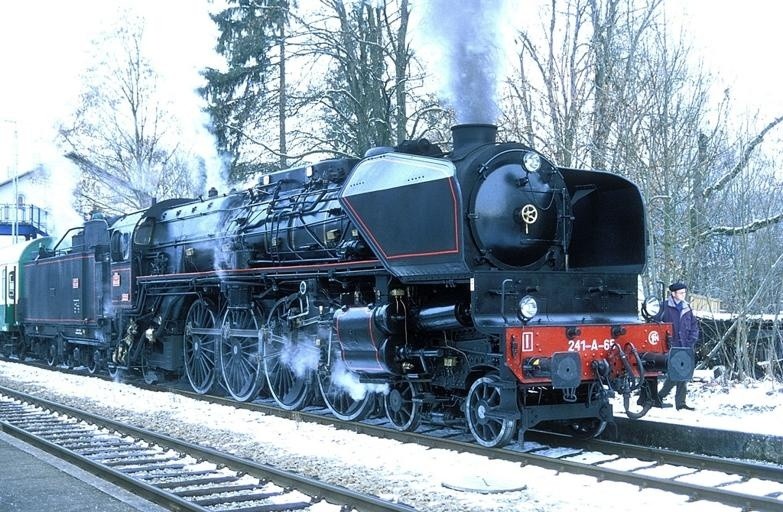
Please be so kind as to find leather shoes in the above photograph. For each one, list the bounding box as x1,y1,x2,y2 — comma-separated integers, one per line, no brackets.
677,405,695,411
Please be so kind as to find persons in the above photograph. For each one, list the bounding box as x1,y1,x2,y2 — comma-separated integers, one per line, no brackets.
653,283,700,411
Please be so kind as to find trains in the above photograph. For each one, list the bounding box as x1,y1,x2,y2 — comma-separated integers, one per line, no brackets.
0,125,696,450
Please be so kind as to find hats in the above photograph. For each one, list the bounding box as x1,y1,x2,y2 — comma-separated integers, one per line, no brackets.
669,283,687,292
